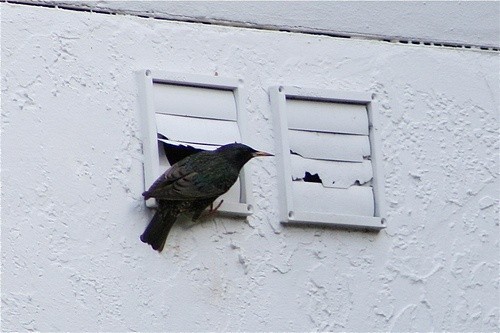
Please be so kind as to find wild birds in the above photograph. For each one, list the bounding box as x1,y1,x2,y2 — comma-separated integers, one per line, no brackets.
140,133,276,253
